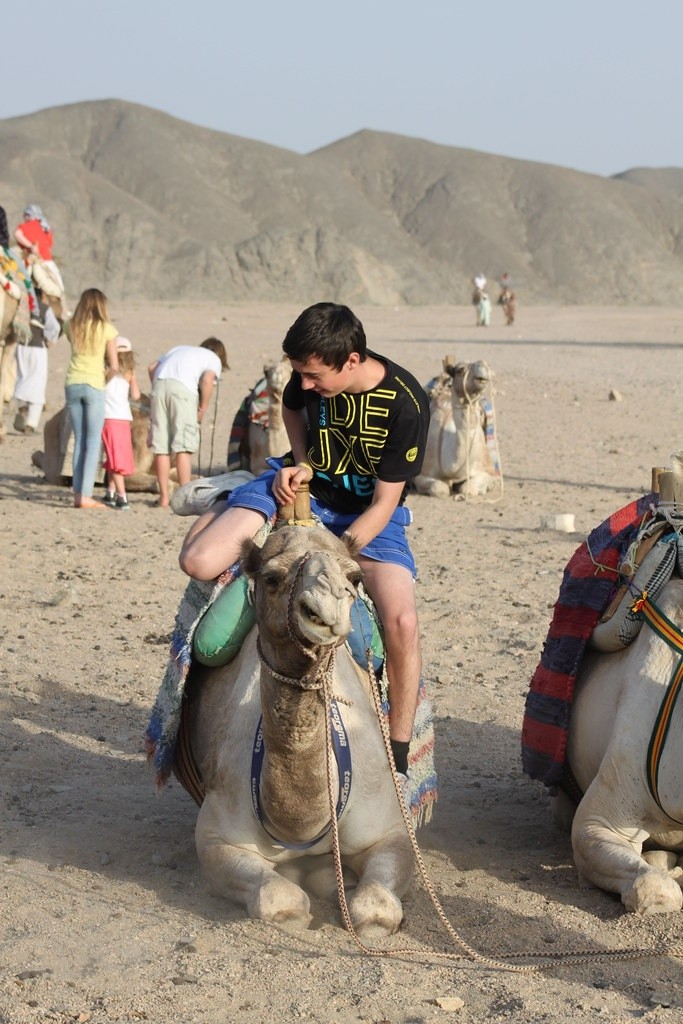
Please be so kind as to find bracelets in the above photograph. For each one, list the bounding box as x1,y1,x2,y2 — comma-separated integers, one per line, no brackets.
298,461,314,484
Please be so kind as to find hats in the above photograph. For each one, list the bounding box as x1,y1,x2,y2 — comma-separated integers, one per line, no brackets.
115,335,132,353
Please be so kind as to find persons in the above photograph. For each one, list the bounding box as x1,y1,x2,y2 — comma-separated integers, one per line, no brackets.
0,205,232,510
471,273,518,326
168,302,430,775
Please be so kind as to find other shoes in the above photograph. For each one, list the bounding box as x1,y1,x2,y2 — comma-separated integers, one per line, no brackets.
100,496,117,507
12,410,25,432
23,426,41,436
116,499,130,512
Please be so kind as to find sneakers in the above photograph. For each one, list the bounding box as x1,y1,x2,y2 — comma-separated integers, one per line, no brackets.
169,469,257,517
395,770,414,809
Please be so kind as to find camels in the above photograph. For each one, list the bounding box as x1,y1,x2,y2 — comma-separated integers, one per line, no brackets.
471,289,517,326
0,248,65,445
412,361,490,501
165,519,416,941
33,391,207,507
242,354,294,477
568,496,683,919
